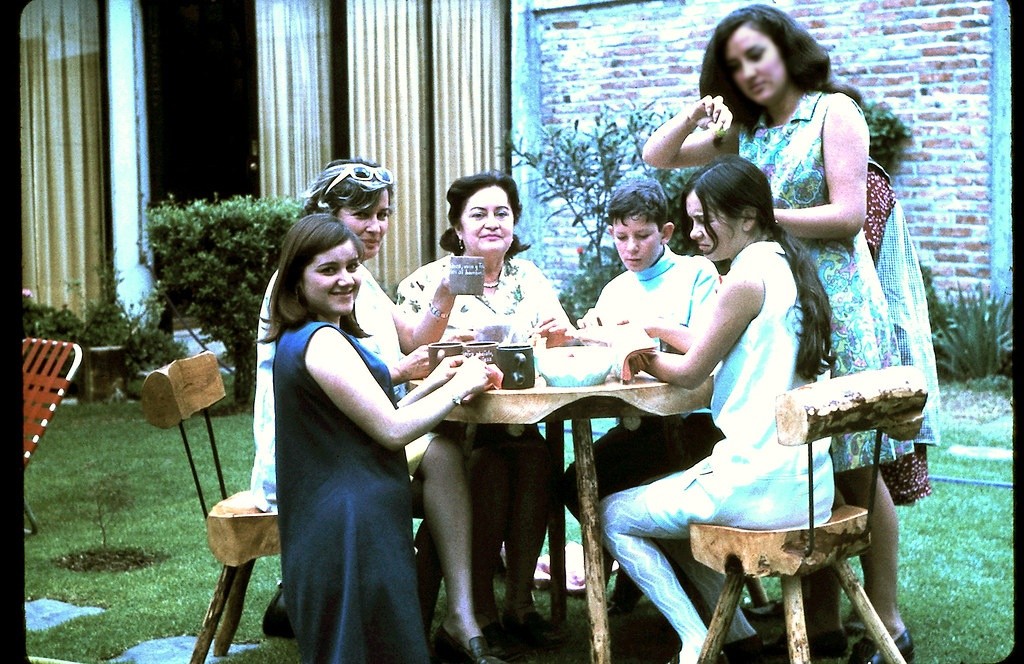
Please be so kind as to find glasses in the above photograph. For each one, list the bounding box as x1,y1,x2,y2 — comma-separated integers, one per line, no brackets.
323,163,395,194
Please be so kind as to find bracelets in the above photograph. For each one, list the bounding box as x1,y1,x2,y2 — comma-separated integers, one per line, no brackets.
443,384,462,404
430,305,450,318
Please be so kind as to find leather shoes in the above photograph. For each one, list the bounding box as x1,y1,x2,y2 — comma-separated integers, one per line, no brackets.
849,630,914,664
432,623,509,664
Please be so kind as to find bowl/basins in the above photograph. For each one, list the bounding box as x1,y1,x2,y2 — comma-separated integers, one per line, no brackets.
536,346,614,386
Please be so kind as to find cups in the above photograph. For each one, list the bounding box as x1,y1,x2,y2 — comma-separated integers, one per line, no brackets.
496,345,536,390
428,341,462,374
448,256,485,296
463,342,498,366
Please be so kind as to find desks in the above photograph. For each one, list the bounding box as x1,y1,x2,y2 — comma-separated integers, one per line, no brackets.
408,371,714,663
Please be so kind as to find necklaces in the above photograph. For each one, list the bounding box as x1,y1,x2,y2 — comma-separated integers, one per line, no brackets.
483,282,497,288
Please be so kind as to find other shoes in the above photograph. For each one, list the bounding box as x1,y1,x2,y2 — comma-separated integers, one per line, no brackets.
503,609,565,649
723,630,765,662
769,628,849,662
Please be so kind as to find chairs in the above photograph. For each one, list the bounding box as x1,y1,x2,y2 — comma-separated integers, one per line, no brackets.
22,337,82,536
141,350,281,664
689,365,928,664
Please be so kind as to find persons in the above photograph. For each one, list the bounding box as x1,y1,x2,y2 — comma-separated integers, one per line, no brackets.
251,159,509,664
393,168,582,661
564,180,721,652
642,4,913,664
599,153,835,664
